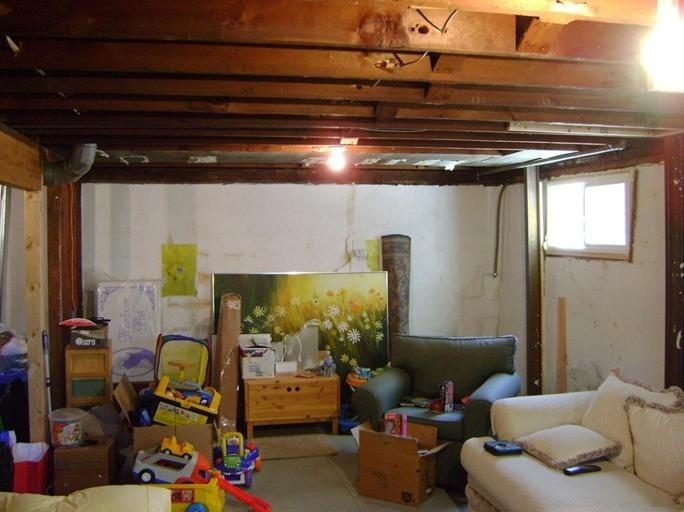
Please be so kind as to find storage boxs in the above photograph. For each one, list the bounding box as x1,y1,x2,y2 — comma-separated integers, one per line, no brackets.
114,373,220,466
71,323,109,347
239,346,274,379
351,419,450,507
48,408,85,447
54,436,116,496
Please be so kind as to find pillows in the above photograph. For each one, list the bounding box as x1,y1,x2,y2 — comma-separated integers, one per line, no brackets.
515,424,622,471
623,394,684,505
579,369,684,469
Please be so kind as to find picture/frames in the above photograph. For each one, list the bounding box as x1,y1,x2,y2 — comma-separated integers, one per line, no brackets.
211,271,389,384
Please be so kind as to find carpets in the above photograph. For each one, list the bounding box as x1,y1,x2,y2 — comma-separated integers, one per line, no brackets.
248,425,338,459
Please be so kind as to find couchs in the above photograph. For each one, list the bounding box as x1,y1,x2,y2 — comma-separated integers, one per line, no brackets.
351,332,521,490
462,370,683,512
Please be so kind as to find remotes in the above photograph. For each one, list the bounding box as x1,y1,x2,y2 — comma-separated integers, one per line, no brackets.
563,464,601,476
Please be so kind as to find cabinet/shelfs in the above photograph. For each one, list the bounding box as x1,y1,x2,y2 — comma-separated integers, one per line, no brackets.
244,370,339,440
65,339,111,408
